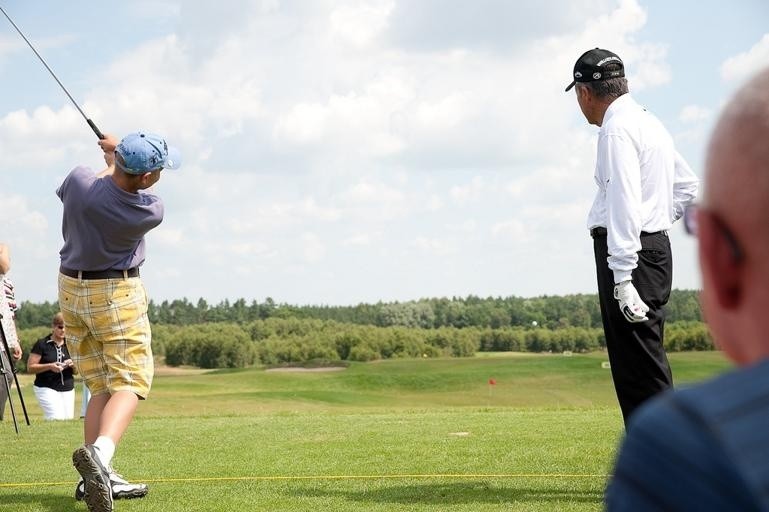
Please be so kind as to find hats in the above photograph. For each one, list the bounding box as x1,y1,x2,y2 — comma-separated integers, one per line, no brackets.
565,48,625,90
113,129,182,175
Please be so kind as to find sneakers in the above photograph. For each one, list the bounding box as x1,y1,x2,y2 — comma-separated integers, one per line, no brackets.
72,445,115,511
76,469,148,501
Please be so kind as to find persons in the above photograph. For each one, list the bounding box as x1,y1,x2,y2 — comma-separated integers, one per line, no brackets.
0,243,24,421
25,311,79,422
594,67,768,512
562,47,699,440
54,129,183,512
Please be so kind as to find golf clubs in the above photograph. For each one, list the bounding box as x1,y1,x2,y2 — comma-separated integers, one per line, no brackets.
533,321,537,326
489,378,496,384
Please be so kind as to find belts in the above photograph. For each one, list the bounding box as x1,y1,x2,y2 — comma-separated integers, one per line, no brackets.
590,228,605,238
58,266,139,279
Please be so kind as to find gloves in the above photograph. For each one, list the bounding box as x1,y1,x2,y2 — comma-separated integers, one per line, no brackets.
614,281,650,322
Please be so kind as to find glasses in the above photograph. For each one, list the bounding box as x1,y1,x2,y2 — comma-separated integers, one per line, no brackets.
57,326,64,329
682,205,742,263
